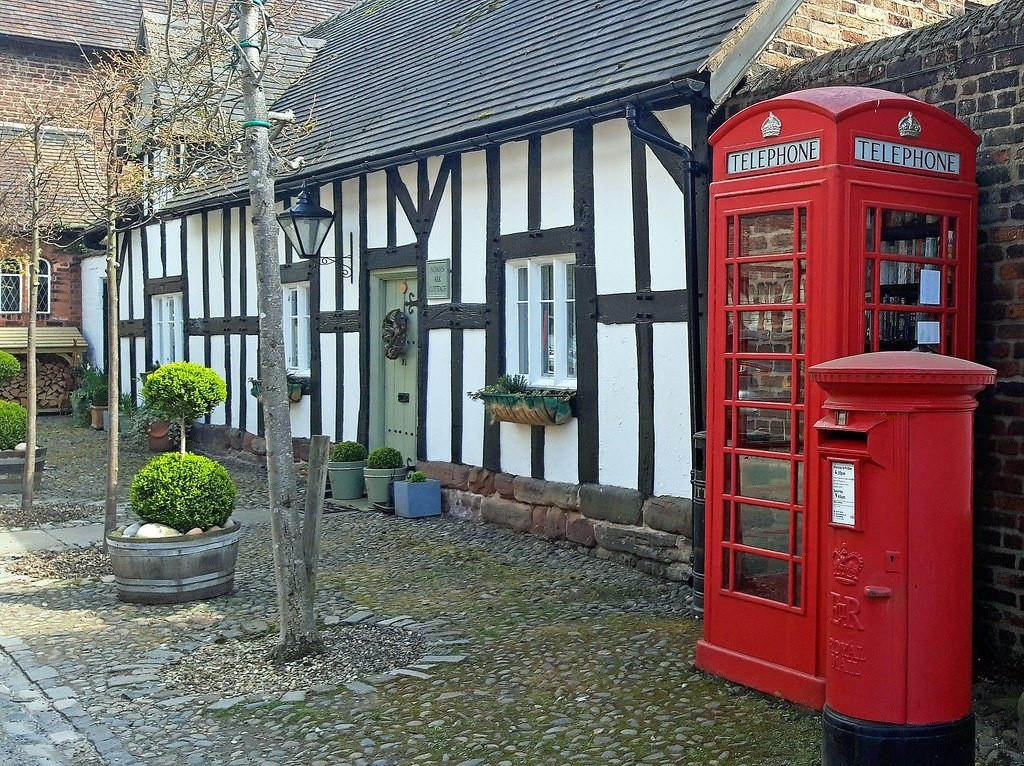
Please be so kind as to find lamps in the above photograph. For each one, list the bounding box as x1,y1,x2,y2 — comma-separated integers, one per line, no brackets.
275,185,353,284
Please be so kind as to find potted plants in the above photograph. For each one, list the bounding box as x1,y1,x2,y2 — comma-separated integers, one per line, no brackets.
394,471,441,518
105,451,240,605
0,400,47,493
76,364,177,450
328,440,367,500
363,446,407,502
